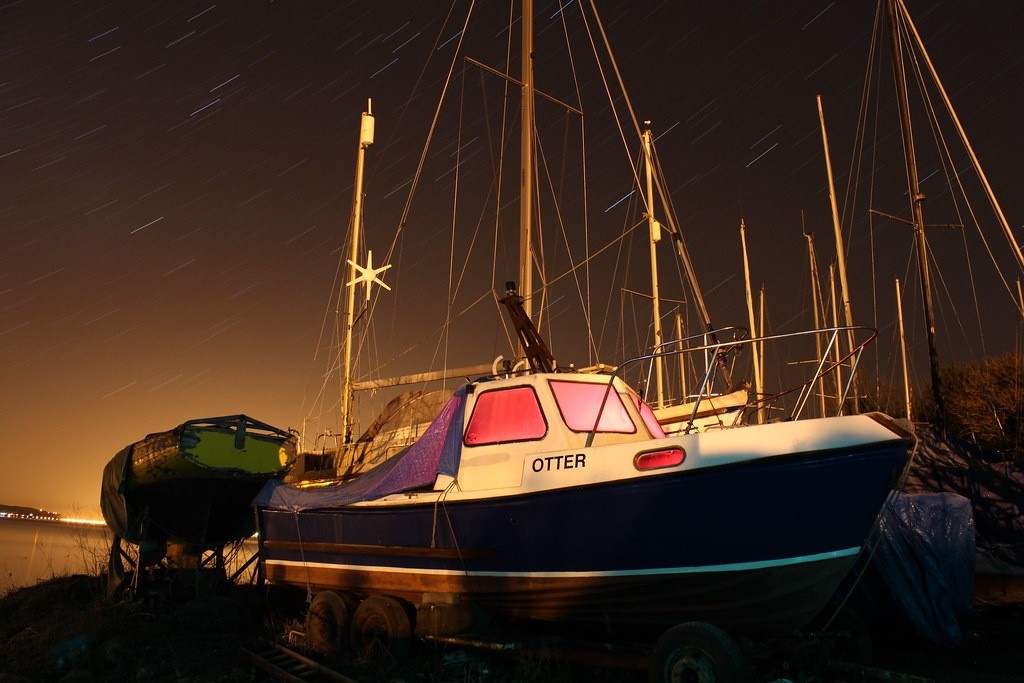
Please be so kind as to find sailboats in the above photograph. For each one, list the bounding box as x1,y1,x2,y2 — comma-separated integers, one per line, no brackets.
97,1,916,640
